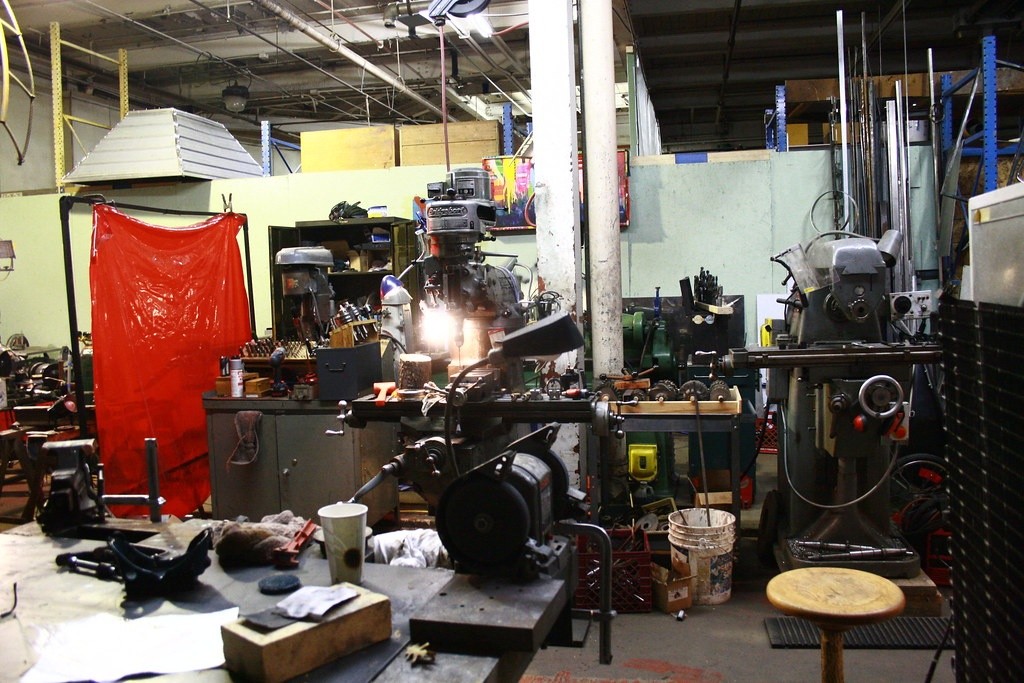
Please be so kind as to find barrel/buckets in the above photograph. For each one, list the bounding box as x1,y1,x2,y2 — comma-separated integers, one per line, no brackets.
667,507,737,606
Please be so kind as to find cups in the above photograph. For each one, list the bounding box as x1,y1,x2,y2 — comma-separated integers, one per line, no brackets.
317,502,368,587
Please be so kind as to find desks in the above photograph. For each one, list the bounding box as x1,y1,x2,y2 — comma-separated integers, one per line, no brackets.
0,520,541,683
351,391,598,522
584,415,744,578
12,346,62,360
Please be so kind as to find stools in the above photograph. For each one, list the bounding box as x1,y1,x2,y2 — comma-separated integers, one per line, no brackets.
766,567,905,683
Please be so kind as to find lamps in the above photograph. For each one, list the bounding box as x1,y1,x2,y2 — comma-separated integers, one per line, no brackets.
222,79,250,112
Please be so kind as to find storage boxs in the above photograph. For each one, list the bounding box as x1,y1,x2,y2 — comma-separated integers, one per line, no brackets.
322,239,350,259
345,250,374,271
694,469,733,511
755,411,778,454
574,528,653,614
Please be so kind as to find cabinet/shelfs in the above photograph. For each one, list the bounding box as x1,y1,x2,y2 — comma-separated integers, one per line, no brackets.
267,217,426,341
202,389,401,528
